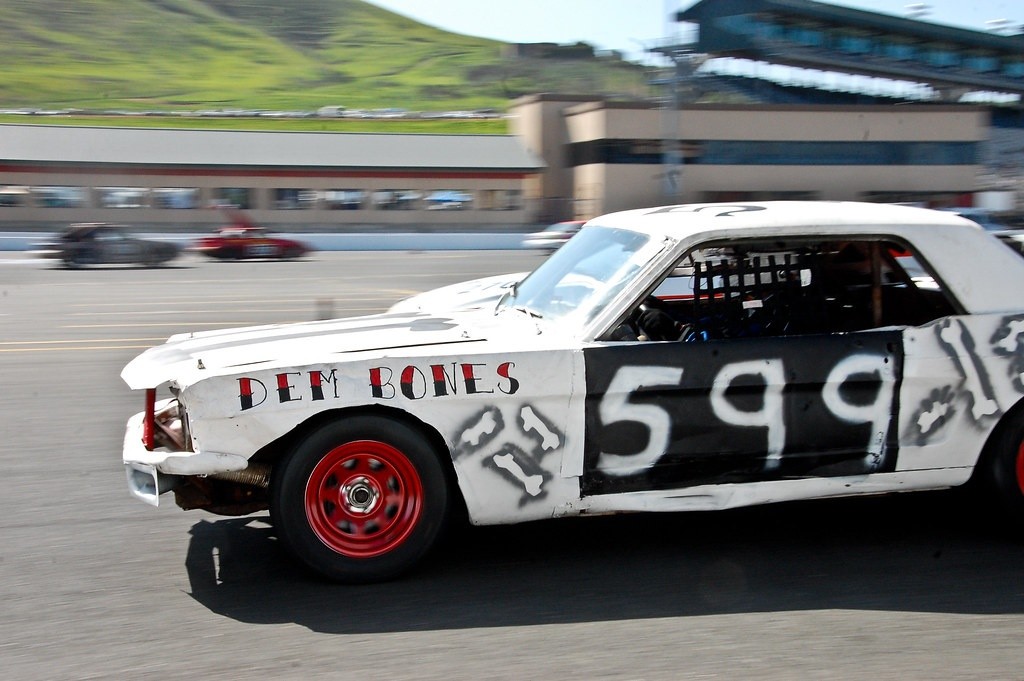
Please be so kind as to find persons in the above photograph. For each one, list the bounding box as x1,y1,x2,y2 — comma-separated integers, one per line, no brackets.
638,242,814,340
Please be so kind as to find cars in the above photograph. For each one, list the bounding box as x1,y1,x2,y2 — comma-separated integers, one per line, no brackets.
26,219,184,268
118,198,1024,590
182,202,313,260
384,205,1024,311
520,219,590,255
